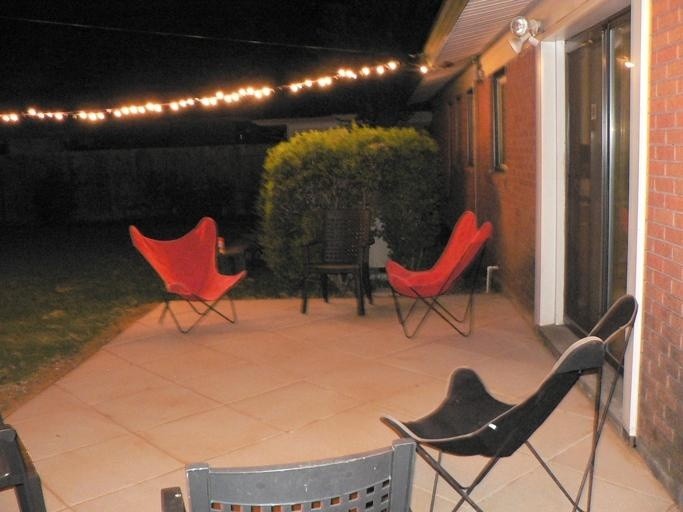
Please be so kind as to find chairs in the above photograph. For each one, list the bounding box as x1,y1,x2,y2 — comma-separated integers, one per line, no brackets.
301,207,373,317
0,415,46,512
379,293,639,512
385,211,494,339
128,217,247,334
161,438,417,512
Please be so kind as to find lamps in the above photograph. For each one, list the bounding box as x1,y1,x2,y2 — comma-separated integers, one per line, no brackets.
506,16,542,55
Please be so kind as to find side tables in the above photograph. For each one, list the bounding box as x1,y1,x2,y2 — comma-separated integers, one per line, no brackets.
214,245,245,275
